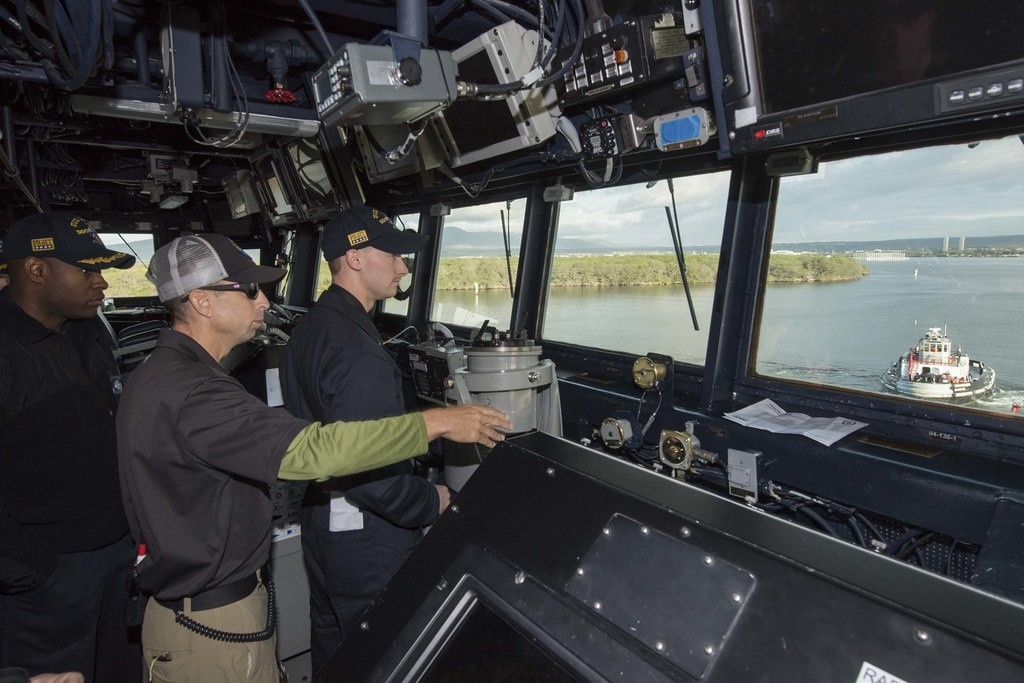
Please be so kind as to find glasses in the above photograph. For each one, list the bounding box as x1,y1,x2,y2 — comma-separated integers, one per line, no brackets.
180,282,259,303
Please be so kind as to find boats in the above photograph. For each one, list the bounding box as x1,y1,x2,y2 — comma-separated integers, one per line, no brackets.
879,320,995,398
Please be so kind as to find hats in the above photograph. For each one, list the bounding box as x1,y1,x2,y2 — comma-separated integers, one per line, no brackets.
1,210,136,272
319,206,431,261
145,231,288,304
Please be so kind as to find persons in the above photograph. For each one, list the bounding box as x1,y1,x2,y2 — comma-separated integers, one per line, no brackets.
116,233,514,683
0,211,136,683
278,206,450,681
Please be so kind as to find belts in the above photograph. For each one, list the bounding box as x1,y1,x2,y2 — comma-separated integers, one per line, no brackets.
153,569,264,612
